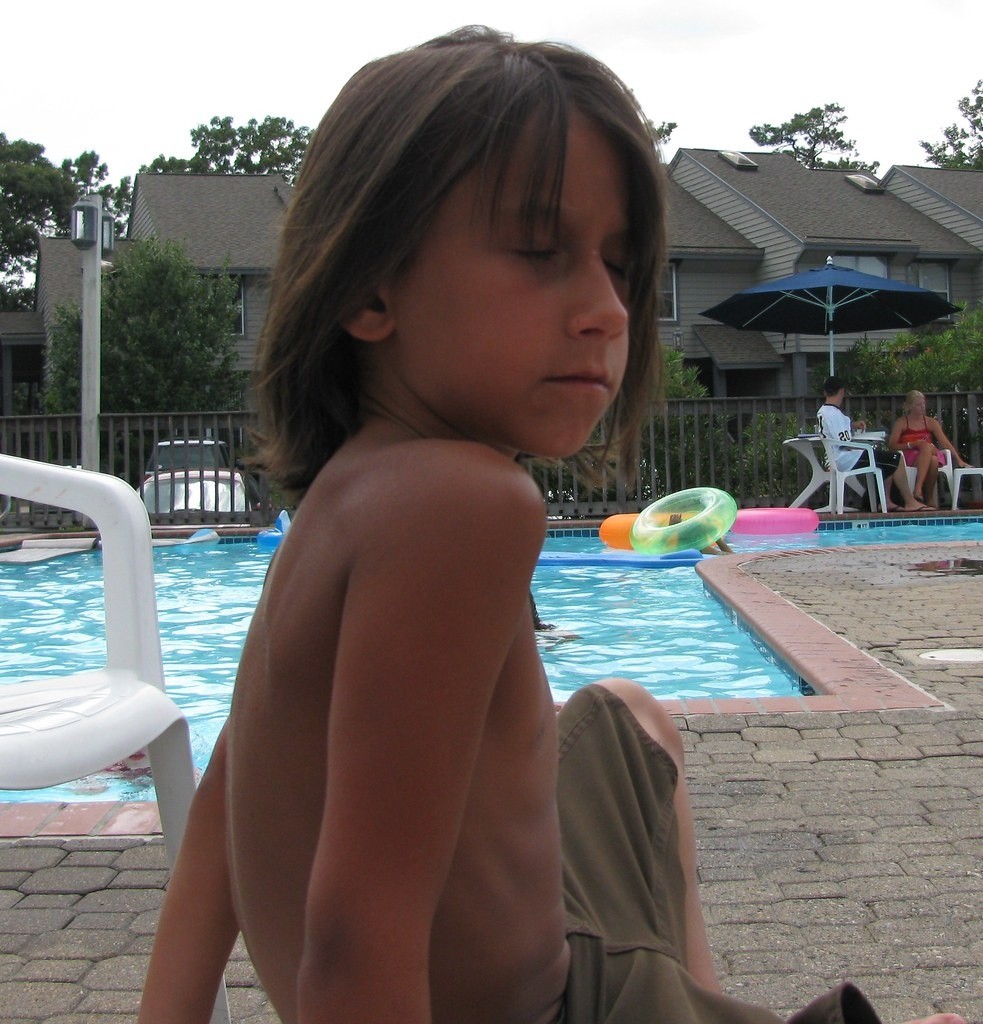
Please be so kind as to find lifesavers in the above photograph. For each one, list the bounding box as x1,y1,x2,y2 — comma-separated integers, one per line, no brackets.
728,508,820,534
598,487,737,555
256,510,292,550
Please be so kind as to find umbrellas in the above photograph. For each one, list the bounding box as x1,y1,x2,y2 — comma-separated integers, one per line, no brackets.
697,256,965,377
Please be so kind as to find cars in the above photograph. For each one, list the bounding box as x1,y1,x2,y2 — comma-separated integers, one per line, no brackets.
135,469,274,530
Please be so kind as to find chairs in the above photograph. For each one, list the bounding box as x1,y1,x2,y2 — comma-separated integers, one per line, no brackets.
898,447,953,499
820,438,887,514
0,448,234,1024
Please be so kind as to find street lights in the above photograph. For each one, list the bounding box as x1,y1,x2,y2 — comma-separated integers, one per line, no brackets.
71,191,118,526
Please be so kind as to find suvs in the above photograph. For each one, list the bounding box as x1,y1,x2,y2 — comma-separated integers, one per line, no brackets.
145,439,244,479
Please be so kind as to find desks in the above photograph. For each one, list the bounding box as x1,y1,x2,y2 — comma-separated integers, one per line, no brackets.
783,437,885,508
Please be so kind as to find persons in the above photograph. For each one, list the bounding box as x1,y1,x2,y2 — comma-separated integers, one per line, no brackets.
817,375,937,511
143,24,965,1024
887,390,974,510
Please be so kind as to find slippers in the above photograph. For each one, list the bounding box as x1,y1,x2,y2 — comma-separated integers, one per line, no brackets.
903,505,937,512
913,490,923,500
878,504,905,512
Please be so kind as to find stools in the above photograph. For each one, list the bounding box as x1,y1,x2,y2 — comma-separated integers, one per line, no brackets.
952,467,983,511
